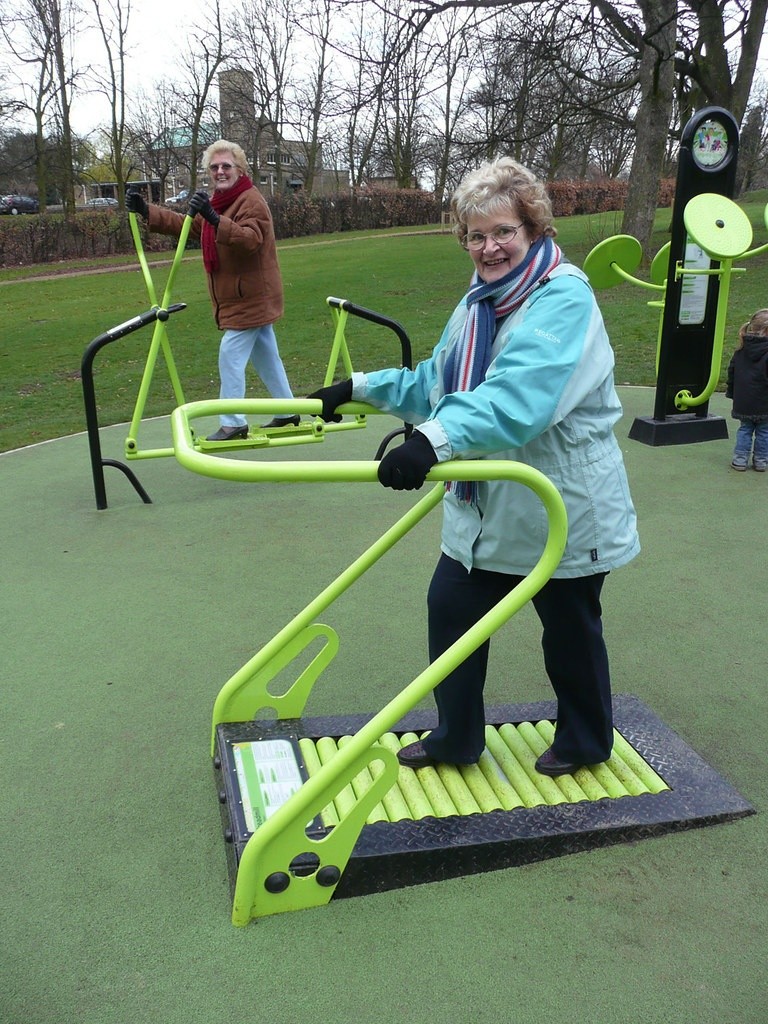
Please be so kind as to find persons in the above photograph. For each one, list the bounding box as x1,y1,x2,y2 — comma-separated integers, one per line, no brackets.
124,140,301,441
308,155,642,775
725,308,768,472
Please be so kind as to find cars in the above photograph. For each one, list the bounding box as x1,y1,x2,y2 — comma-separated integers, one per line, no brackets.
86,198,117,205
0,194,40,216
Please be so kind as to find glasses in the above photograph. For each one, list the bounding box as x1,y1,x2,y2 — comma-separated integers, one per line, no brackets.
461,222,526,251
209,163,238,172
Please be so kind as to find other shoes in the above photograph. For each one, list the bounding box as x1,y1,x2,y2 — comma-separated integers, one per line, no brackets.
732,463,747,471
535,745,581,775
396,739,485,766
756,466,765,472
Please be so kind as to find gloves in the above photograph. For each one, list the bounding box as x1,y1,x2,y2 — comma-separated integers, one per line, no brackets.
307,377,353,422
188,191,219,225
124,189,148,219
378,432,437,491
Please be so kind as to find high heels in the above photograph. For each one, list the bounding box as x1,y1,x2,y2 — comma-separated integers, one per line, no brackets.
261,414,300,428
206,424,249,441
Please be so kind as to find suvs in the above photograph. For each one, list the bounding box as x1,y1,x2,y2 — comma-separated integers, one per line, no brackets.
165,189,209,203
